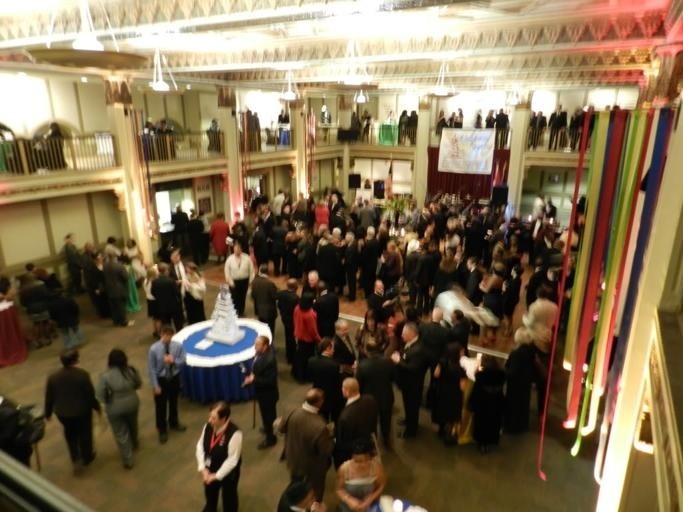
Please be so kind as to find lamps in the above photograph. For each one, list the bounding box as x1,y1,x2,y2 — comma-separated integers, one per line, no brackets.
72,0,103,51
281,71,299,101
353,86,372,106
428,64,458,97
148,50,172,93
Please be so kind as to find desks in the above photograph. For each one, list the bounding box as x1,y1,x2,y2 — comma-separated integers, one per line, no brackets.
167,316,267,407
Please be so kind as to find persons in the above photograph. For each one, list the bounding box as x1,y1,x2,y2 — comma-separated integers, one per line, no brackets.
435,107,508,148
145,120,173,134
251,112,262,152
350,110,372,144
527,104,620,153
397,108,418,146
277,109,290,145
194,376,386,510
210,120,217,131
170,190,621,453
45,123,62,138
0,232,206,476
